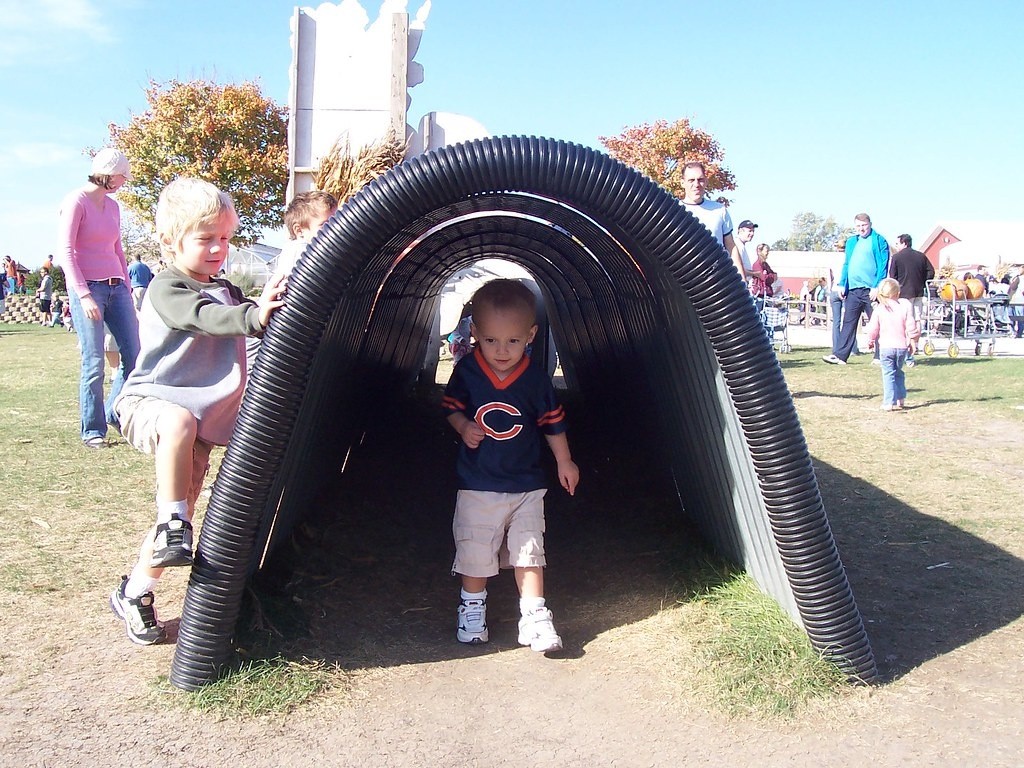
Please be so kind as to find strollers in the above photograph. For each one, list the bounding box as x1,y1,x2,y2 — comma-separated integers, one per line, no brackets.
760,287,792,355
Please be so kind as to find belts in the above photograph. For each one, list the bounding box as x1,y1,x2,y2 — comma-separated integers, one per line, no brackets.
100,277,123,286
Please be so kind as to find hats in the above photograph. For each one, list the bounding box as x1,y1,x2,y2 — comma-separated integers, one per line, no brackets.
738,219,758,229
93,149,136,183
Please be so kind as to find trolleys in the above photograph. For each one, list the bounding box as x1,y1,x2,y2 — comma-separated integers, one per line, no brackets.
923,279,1002,358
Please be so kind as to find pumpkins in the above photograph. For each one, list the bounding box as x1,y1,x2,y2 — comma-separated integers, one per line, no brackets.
964,279,984,299
940,278,969,300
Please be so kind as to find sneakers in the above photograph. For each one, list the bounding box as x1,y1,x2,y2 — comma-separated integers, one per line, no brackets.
822,354,846,365
109,574,168,646
150,511,195,568
517,597,562,651
871,358,880,365
456,588,489,644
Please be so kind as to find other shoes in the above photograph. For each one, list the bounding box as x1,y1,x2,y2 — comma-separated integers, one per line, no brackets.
87,438,106,449
897,399,903,407
879,403,891,410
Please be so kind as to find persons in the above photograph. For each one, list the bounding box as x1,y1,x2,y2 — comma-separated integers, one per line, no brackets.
800,278,827,326
889,234,935,355
937,264,1024,338
822,213,890,365
114,177,338,646
866,278,919,411
678,163,783,300
442,279,579,651
0,255,73,333
60,147,154,449
